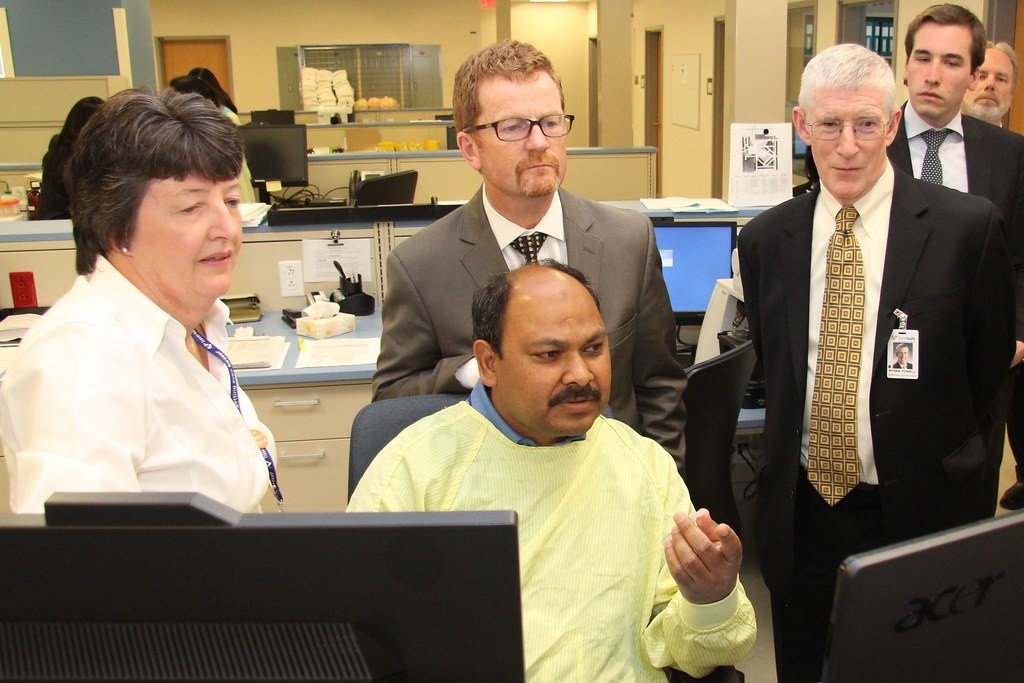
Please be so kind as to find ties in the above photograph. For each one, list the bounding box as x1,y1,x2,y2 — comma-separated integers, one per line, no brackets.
806,207,865,506
509,233,547,265
921,130,949,189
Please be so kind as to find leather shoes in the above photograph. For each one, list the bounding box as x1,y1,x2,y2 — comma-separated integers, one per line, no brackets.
999,481,1024,510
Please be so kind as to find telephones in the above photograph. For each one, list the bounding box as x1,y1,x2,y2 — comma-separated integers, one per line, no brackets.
350,169,385,199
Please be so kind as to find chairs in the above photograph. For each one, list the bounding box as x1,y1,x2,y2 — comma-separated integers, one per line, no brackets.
684,340,758,537
349,394,614,511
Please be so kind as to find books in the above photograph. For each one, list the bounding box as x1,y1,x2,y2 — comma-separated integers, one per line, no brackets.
0,314,43,343
227,336,284,368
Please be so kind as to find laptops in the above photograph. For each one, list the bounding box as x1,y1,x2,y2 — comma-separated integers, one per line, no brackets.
819,508,1023,683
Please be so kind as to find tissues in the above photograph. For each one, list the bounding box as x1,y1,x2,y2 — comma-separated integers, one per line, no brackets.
296,300,356,340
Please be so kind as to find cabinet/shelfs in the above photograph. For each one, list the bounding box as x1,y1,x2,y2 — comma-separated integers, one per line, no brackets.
238,379,373,513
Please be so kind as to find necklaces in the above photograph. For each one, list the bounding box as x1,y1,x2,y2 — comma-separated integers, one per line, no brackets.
185,329,208,369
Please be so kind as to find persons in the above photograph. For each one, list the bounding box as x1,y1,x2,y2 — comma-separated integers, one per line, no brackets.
35,68,256,220
738,44,1024,683
893,344,912,368
371,40,686,477
346,258,757,683
0,84,278,514
885,4,1024,221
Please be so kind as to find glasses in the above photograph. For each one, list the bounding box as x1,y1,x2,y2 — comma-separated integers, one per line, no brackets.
807,110,892,141
462,112,575,143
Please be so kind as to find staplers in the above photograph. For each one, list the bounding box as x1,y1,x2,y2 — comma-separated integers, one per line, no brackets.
281,308,302,329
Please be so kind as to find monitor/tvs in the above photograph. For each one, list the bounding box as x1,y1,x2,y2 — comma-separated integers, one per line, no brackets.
0,491,524,683
250,110,294,124
237,123,308,205
435,115,454,120
653,220,737,325
447,126,459,149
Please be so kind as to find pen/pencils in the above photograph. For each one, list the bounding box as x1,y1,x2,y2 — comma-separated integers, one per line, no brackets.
333,261,347,280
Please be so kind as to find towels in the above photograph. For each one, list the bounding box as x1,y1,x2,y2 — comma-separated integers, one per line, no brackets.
300,66,356,110
